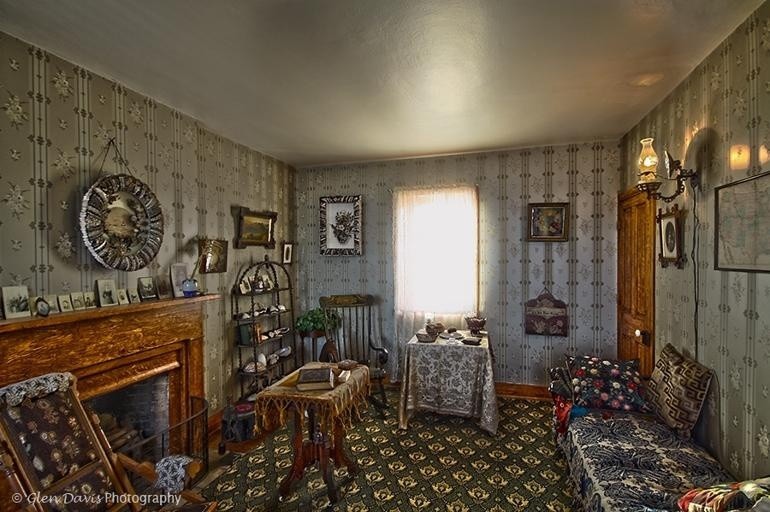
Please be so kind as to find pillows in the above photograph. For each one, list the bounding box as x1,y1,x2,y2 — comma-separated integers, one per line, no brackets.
547,340,716,435
659,471,770,512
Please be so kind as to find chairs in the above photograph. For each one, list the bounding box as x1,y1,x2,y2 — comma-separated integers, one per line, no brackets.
0,372,218,511
318,294,390,421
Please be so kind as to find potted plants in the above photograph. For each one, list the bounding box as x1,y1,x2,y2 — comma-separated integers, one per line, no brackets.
294,306,340,338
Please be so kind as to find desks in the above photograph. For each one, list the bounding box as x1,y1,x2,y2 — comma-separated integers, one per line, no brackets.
406,327,490,419
260,360,369,500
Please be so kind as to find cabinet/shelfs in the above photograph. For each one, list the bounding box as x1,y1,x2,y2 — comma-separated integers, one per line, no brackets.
232,254,299,399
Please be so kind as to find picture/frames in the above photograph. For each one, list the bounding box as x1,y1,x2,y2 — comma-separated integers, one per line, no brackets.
317,193,363,256
237,206,295,266
527,201,570,242
655,203,686,270
713,172,769,274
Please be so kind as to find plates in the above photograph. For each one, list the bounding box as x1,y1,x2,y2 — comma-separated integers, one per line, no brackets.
440,332,464,338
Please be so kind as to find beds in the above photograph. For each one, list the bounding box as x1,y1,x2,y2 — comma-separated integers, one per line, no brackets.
548,375,757,511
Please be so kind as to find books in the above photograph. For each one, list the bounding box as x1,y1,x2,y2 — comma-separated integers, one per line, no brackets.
295,367,335,393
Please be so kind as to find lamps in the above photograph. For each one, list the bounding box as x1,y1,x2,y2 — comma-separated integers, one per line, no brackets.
636,137,699,203
